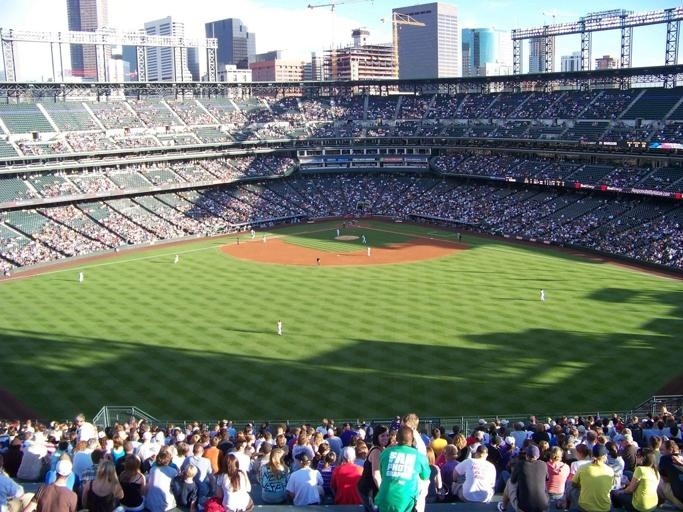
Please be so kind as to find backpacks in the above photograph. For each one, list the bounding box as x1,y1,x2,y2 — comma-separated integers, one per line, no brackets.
197,482,226,512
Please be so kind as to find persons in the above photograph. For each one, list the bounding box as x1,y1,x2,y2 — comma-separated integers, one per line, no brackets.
1,403,683,512
0,89,683,279
539,288,545,301
79,271,84,282
276,319,283,335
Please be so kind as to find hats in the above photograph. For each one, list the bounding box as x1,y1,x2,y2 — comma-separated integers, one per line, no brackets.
474,431,485,440
141,431,152,440
505,436,515,445
176,433,185,441
443,445,459,455
587,430,598,439
295,452,310,463
592,445,609,456
156,432,164,440
525,445,540,458
344,447,356,459
611,418,618,423
618,434,633,442
98,431,106,438
500,420,509,425
56,460,72,476
479,419,487,424
